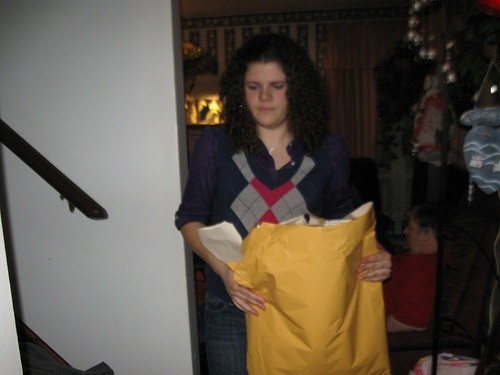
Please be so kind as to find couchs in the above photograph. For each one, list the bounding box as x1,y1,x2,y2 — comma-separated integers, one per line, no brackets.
388,201,500,375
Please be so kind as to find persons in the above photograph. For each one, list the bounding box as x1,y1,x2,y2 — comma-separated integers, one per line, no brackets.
174,33,393,375
383,204,439,332
